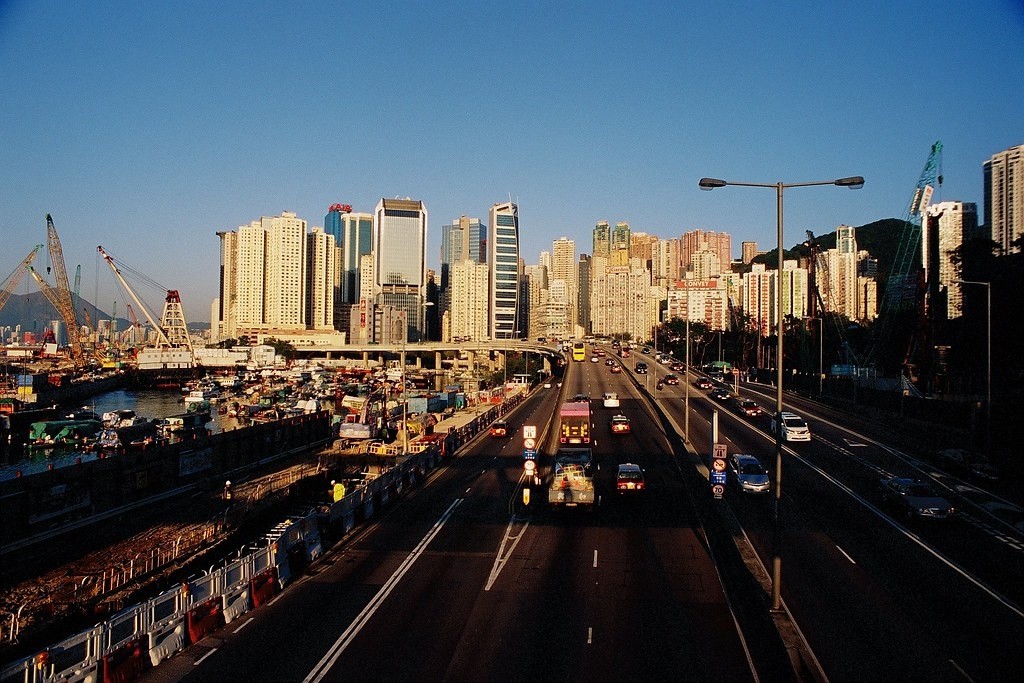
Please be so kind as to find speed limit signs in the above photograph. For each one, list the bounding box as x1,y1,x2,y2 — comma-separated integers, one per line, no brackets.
524,461,535,470
713,484,724,494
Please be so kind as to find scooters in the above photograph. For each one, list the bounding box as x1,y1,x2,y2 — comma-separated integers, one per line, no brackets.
657,383,663,390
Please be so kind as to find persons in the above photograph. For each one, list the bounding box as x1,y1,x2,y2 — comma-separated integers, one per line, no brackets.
36,646,56,682
331,480,345,502
222,481,234,499
270,540,278,564
310,505,330,514
146,435,152,442
182,580,189,599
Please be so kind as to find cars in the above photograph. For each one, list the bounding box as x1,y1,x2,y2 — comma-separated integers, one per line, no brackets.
544,341,650,374
613,463,646,496
664,374,678,384
736,399,762,417
712,388,730,400
610,415,631,435
881,479,956,524
490,419,509,436
654,351,671,364
602,392,620,407
671,362,682,370
695,379,713,389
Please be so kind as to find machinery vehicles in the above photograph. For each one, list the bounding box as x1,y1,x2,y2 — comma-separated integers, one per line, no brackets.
339,385,387,442
806,140,944,402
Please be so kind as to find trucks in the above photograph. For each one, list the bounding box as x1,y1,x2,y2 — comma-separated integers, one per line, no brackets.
548,394,595,510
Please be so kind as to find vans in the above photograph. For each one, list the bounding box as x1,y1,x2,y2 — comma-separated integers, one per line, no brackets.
771,412,811,442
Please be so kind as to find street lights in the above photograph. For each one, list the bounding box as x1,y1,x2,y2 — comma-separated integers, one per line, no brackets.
475,324,500,417
401,302,434,455
504,331,521,399
699,176,866,609
654,274,721,444
951,279,991,406
801,316,823,395
747,324,760,369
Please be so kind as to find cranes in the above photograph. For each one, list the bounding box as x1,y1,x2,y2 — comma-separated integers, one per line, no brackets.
96,245,191,350
0,213,145,366
